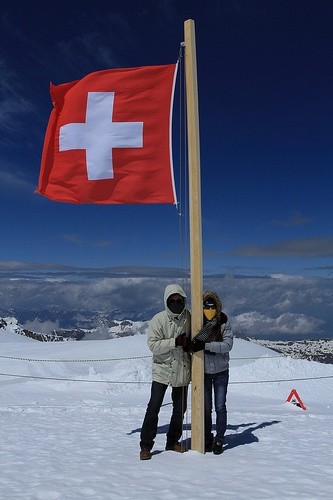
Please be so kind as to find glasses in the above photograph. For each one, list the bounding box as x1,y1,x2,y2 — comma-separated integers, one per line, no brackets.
166,298,184,304
204,303,217,309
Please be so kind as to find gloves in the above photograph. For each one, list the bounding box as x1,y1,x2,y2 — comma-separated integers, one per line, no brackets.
175,333,188,346
190,339,205,352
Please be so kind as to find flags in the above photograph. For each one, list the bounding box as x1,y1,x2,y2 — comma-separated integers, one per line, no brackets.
34,64,179,204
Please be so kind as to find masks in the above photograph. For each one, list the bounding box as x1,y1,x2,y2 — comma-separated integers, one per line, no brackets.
168,304,185,314
203,308,217,320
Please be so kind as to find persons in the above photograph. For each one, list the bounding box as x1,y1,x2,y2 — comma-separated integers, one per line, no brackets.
193,291,233,455
140,284,192,460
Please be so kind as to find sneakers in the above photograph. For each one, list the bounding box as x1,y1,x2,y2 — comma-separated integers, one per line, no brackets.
166,441,188,452
140,446,151,460
205,433,214,451
212,441,223,454
